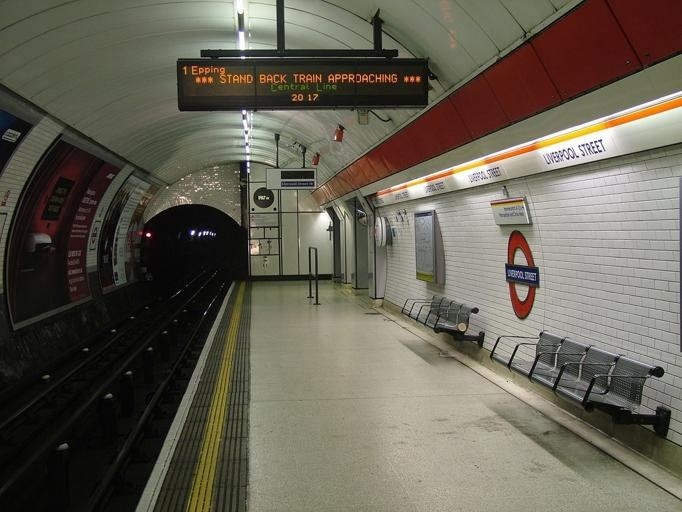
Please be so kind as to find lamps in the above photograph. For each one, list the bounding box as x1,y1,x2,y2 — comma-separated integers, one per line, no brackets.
311,152,321,165
236,1,251,175
330,123,345,142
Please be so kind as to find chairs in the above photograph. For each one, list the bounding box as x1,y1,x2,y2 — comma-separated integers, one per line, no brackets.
402,293,443,325
529,344,619,395
489,329,565,372
505,337,590,382
409,295,451,318
417,300,456,327
425,303,485,349
554,356,671,437
